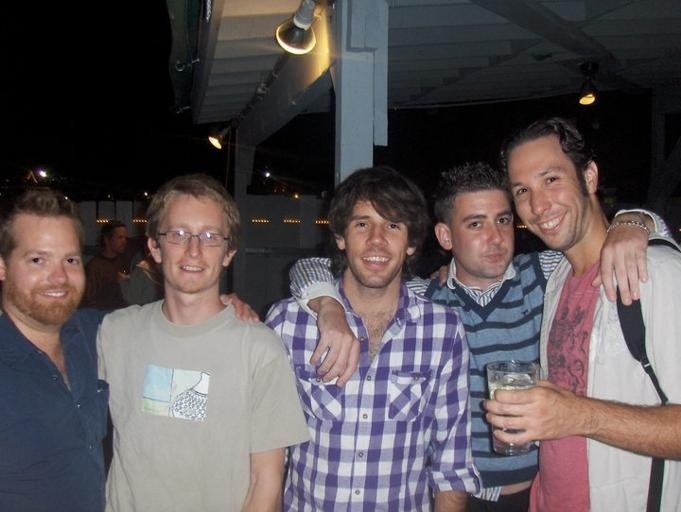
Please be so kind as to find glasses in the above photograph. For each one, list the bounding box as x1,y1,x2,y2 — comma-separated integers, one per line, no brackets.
156,229,231,247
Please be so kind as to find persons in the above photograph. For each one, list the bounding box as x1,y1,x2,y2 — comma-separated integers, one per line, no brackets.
289,161,673,512
86,220,127,311
95,172,311,512
428,118,681,511
0,186,262,511
116,240,163,307
265,166,482,512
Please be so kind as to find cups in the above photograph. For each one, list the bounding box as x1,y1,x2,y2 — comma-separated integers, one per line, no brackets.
486,360,540,456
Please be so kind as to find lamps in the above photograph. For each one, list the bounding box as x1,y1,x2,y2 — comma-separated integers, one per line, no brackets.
578,63,598,105
176,59,199,71
208,127,230,150
275,0,316,55
174,104,190,114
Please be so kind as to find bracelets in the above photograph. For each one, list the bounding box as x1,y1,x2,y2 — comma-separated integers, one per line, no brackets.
607,220,650,233
